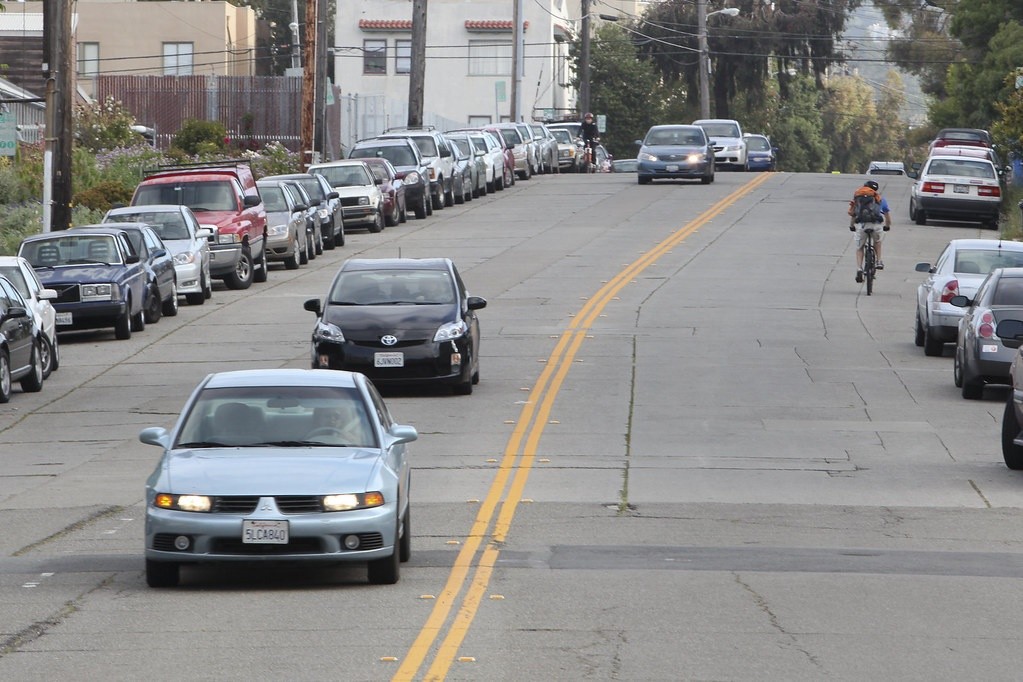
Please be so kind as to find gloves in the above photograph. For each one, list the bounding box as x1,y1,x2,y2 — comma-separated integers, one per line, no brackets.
849,225,855,232
883,225,890,231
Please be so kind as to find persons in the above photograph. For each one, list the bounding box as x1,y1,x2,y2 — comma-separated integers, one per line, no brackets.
311,407,342,436
413,277,451,301
850,181,891,282
576,113,600,168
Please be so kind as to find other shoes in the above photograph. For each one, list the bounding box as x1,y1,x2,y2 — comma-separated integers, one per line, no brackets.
876,260,884,269
855,269,864,283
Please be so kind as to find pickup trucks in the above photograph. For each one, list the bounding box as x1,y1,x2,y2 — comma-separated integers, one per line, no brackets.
128,158,268,289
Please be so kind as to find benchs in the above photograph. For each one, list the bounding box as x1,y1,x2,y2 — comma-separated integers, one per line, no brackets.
956,260,979,273
206,410,313,438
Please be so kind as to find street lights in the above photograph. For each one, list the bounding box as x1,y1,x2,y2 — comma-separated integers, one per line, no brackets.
698,8,740,121
289,22,301,69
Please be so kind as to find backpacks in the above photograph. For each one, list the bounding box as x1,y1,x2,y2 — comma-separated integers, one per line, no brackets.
854,195,884,223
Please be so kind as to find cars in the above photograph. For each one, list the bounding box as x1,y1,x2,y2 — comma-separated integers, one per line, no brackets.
915,239,1022,357
0,256,59,370
949,267,1023,400
303,257,488,396
255,157,408,269
17,227,148,341
634,119,779,186
996,319,1023,470
0,273,44,404
100,204,212,306
866,129,1013,231
138,368,418,588
47,221,179,324
442,122,638,205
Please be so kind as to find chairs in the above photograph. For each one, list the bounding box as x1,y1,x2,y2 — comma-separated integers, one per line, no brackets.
417,143,432,153
348,173,363,187
263,191,285,212
311,406,352,435
165,216,185,238
38,245,60,266
88,239,111,261
214,402,257,441
348,277,380,300
392,149,407,165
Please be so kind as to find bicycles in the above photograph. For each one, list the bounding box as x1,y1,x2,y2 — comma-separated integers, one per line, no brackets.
576,138,597,174
852,229,889,296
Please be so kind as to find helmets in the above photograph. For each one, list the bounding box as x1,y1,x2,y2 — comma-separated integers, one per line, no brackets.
865,181,878,190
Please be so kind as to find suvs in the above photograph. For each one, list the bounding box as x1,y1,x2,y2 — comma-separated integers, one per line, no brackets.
347,126,455,219
